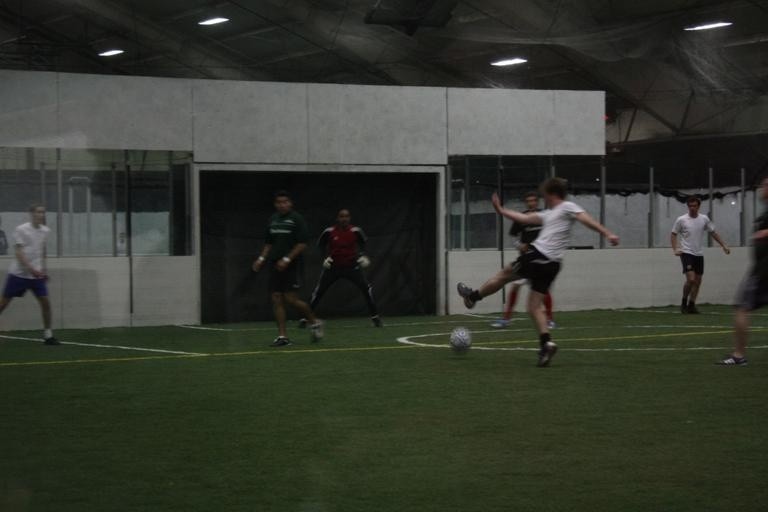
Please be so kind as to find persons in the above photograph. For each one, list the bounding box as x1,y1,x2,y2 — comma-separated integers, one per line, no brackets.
249,191,324,348
457,175,620,367
490,192,557,332
715,174,768,364
295,203,384,331
0,202,60,346
669,196,732,314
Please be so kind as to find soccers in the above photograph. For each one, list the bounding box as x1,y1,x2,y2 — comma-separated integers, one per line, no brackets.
451,328,473,348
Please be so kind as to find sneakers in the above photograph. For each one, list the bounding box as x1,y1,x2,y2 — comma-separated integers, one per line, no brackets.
713,354,747,366
371,314,383,326
300,316,323,339
680,304,699,314
457,282,475,308
268,336,292,347
43,336,60,346
538,341,557,367
491,318,510,328
